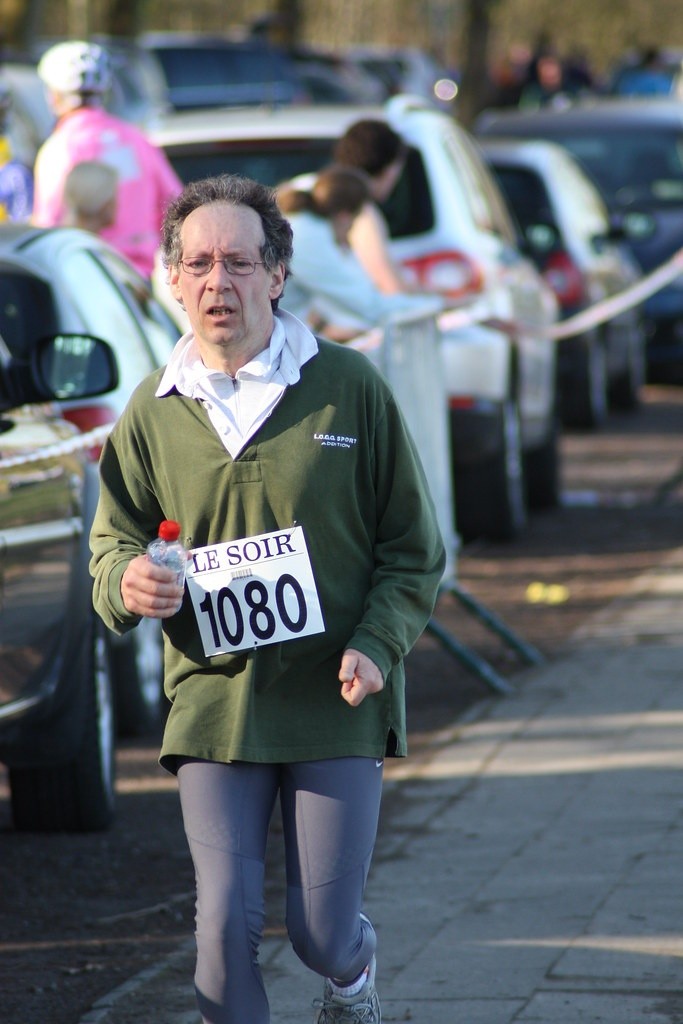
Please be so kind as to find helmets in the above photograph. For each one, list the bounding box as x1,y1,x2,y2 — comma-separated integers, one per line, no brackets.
36,39,114,92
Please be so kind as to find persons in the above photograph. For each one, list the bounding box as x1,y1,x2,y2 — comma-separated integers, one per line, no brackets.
0,41,189,278
518,44,672,116
273,119,445,346
89,175,448,1024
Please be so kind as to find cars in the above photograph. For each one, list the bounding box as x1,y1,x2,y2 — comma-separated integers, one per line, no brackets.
0,224,191,838
0,27,683,595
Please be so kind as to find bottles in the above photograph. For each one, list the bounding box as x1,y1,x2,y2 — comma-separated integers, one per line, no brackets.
147,520,188,613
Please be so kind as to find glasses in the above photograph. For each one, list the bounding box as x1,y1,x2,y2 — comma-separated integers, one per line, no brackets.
178,256,265,275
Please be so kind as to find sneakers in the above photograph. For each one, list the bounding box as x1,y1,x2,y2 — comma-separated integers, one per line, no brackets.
311,911,381,1024
